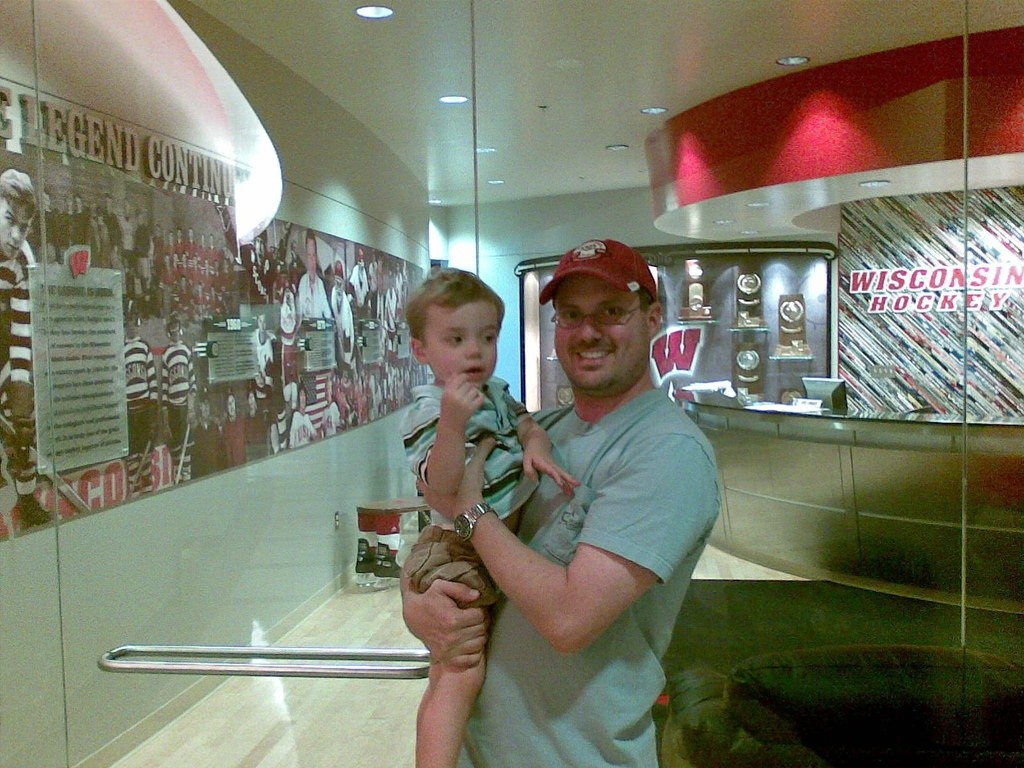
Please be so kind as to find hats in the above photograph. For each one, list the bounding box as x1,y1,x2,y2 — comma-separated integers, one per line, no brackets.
226,388,235,399
539,240,657,305
247,383,256,396
199,387,210,402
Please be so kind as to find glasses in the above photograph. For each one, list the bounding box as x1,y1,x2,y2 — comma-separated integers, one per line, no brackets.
551,302,642,330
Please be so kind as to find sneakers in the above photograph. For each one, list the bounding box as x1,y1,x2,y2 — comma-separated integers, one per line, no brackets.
354,538,377,585
373,541,403,589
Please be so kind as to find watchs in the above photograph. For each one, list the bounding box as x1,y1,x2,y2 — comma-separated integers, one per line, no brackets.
452,502,499,541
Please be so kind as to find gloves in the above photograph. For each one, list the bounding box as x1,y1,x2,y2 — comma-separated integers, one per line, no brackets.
186,408,197,424
162,423,172,441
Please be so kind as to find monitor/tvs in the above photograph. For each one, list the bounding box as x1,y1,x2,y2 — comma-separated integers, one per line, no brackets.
801,376,849,411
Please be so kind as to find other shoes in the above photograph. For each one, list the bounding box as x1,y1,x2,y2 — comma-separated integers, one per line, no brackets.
17,492,48,524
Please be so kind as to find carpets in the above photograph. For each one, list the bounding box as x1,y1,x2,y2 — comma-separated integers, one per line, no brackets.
652,580,1024,768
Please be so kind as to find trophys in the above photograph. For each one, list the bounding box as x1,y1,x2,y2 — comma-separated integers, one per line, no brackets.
551,260,816,411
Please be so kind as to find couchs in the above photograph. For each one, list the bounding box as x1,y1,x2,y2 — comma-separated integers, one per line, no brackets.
660,644,1024,768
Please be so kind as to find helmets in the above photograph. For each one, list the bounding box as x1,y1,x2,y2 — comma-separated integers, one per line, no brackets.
123,311,142,327
334,261,343,283
358,249,364,261
254,231,267,247
165,311,182,342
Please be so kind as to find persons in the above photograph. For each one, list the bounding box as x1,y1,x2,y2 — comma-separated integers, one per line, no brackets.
242,230,424,456
400,268,581,768
398,240,723,768
0,169,249,525
